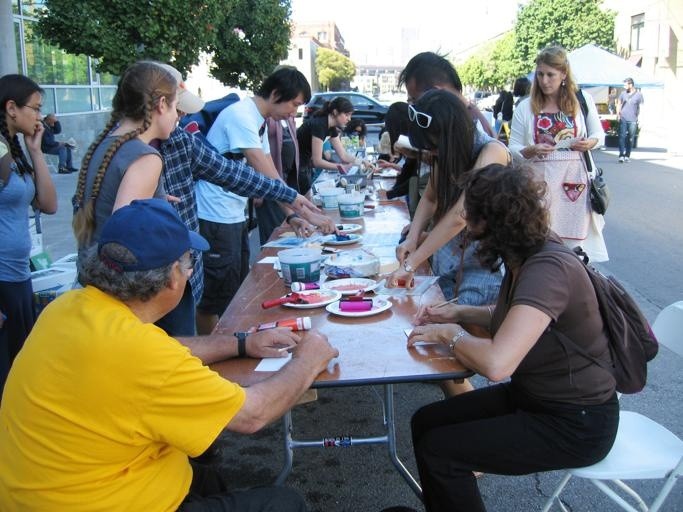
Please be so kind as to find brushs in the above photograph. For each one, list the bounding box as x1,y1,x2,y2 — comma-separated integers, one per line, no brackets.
331,229,352,241
338,287,373,312
261,290,309,309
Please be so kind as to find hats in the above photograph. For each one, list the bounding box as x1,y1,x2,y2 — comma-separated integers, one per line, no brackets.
156,63,205,114
99,199,210,271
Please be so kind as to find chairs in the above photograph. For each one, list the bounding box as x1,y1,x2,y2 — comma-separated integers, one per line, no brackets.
536,297,681,512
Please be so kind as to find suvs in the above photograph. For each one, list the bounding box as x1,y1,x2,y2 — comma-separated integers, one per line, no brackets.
300,88,395,128
468,89,492,106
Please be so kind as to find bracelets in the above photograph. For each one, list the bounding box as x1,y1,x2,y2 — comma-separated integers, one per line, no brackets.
232,331,249,358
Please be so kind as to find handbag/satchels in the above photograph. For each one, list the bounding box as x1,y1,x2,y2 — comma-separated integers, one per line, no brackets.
590,174,609,215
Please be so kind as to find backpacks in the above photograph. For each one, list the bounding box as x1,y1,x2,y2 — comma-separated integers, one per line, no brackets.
525,243,658,394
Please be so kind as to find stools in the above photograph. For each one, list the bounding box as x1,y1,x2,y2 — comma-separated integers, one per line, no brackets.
40,150,59,173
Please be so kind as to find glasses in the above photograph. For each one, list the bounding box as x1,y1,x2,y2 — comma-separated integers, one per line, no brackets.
408,104,434,128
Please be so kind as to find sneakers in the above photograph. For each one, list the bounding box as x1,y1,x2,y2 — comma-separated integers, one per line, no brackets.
59,167,78,173
619,157,629,161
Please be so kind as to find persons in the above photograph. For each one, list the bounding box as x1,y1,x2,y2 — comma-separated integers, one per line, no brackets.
40,114,78,174
70,46,619,509
0,198,340,510
616,78,644,163
0,73,57,403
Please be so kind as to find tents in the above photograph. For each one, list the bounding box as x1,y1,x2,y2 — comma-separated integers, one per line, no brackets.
527,45,664,87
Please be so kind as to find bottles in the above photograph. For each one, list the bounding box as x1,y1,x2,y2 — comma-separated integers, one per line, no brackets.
351,131,360,148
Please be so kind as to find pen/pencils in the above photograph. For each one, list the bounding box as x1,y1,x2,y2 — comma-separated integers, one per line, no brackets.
431,296,459,310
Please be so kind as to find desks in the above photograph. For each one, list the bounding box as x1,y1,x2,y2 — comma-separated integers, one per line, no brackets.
203,227,480,507
280,143,416,233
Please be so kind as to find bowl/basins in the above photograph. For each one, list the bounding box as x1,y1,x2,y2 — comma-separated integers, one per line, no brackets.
323,249,381,280
275,248,323,289
318,186,344,211
337,192,368,220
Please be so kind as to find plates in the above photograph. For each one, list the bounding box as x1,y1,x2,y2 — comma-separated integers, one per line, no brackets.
278,288,340,310
332,223,363,232
320,277,376,294
326,298,393,318
316,233,363,246
